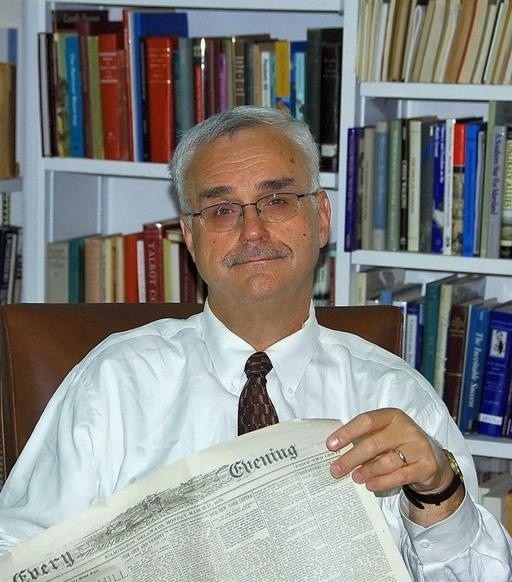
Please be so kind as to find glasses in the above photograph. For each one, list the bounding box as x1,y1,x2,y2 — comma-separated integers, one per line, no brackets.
188,192,314,234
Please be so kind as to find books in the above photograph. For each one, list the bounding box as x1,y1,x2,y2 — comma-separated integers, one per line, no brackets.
45,217,209,303
351,268,512,439
344,102,512,260
477,473,512,539
311,242,336,307
355,2,512,86
0,28,26,304
36,1,342,173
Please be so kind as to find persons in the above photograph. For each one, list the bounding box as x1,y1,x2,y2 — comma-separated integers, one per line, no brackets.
0,103,512,582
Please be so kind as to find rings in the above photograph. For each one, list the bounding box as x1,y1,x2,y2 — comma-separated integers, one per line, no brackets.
392,447,410,468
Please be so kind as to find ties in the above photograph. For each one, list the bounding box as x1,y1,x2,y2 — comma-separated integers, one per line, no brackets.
235,349,279,436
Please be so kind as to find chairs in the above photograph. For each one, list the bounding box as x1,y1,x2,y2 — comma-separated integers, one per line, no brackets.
0,303,405,493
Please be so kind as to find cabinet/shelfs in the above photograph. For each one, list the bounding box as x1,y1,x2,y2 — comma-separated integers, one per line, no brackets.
351,0,512,471
19,0,360,307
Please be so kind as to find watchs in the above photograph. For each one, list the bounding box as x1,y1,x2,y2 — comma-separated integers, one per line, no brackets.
400,447,464,511
